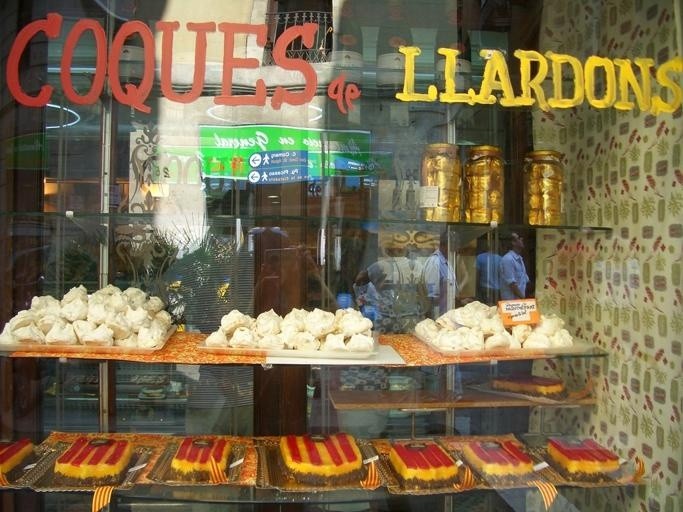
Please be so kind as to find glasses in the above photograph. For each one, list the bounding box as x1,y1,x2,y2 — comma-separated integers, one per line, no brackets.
245,226,289,242
513,235,520,242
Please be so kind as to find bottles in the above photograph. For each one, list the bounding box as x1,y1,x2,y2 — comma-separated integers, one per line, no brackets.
520,149,567,229
433,1,472,96
463,142,508,227
417,142,464,224
329,1,364,99
374,1,413,100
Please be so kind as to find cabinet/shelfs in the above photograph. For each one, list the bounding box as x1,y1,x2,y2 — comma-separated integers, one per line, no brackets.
0,69,649,512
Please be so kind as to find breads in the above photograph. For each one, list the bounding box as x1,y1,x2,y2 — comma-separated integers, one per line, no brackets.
1,284,173,350
206,307,376,354
414,300,573,353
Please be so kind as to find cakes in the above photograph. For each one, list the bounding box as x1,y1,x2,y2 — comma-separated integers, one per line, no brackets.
275,432,367,486
171,435,236,483
1,437,40,485
387,441,459,490
53,436,133,488
544,435,622,482
460,440,539,485
491,373,571,400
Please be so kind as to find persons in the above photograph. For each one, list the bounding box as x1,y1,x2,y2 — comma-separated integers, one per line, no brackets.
198,230,531,436
